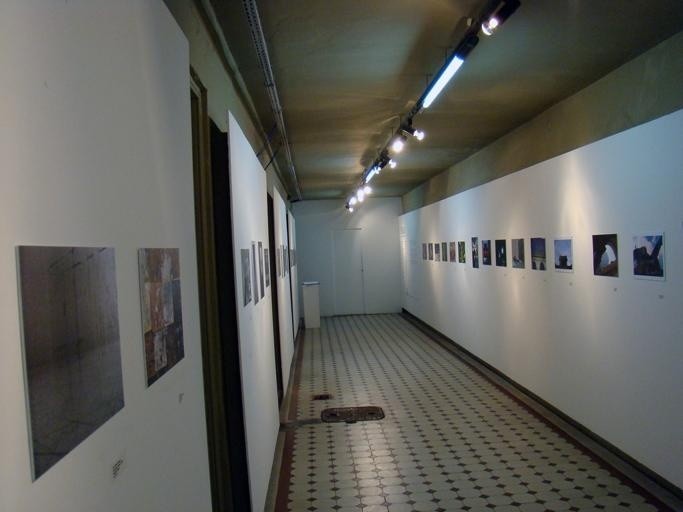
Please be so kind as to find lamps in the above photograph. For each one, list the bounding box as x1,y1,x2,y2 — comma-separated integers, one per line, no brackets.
344,0,523,213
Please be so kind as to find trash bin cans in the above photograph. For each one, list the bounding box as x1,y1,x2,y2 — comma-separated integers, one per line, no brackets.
301,281,320,328
